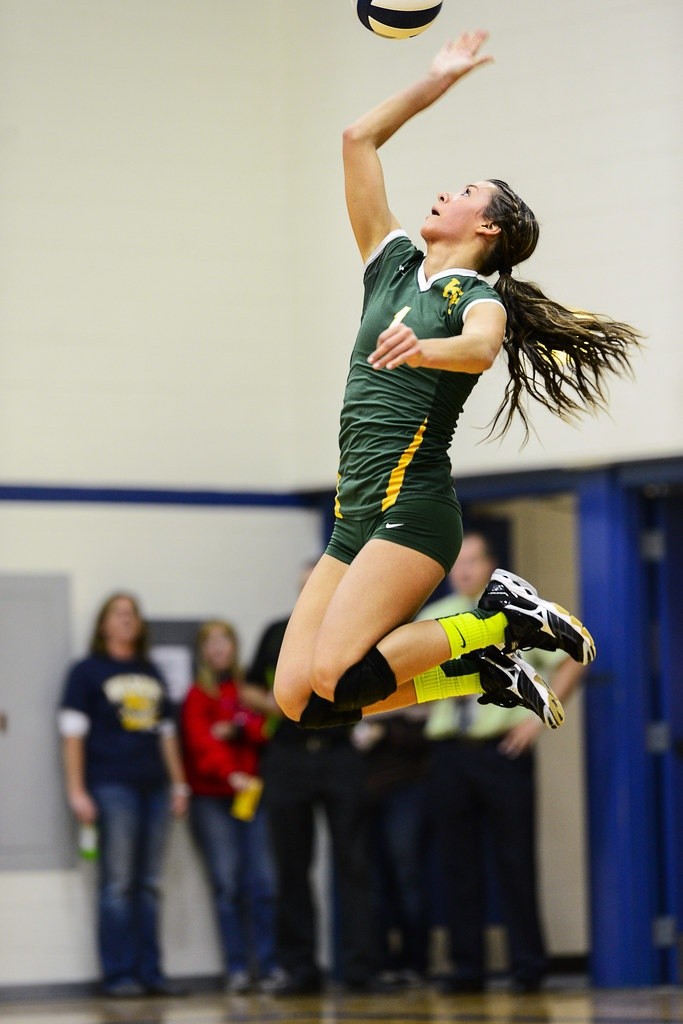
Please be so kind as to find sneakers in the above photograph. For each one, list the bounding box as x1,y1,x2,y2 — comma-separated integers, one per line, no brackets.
462,644,566,731
479,567,596,665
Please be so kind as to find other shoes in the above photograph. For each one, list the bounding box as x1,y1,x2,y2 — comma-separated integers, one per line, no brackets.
389,968,432,988
343,972,405,997
443,973,489,995
272,965,328,996
218,972,264,996
504,968,545,993
93,979,142,997
131,973,185,997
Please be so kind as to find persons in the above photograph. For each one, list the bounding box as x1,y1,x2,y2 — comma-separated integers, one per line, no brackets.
175,618,282,1000
55,591,191,1000
243,534,598,999
272,28,647,734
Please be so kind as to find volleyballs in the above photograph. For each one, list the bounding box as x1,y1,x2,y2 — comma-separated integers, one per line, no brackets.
349,0,445,42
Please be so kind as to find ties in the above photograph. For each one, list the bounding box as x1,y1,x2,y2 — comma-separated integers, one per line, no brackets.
454,695,476,732
